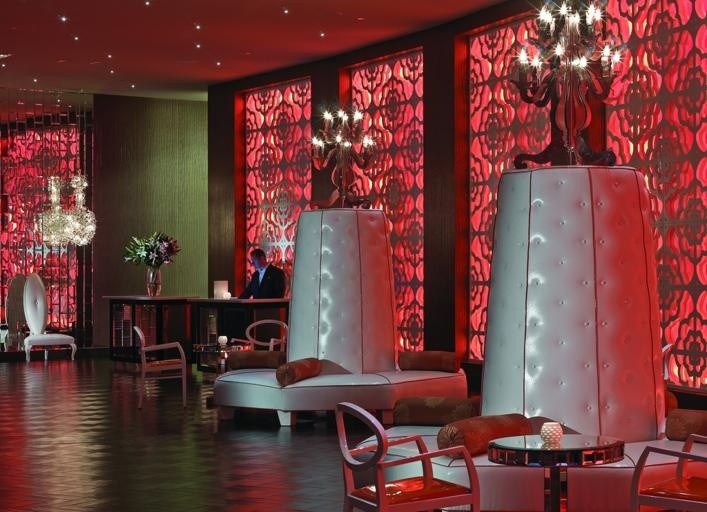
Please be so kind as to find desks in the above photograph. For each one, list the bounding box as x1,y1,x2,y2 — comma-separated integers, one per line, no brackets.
185,294,290,381
98,293,200,373
488,430,625,511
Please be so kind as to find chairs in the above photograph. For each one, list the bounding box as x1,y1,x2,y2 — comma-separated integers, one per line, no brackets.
0,273,26,355
317,397,482,512
629,431,707,512
22,271,76,362
218,320,289,375
125,322,192,411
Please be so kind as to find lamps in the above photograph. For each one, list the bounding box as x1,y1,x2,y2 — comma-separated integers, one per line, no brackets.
36,174,66,248
58,173,98,247
313,106,374,209
503,0,621,169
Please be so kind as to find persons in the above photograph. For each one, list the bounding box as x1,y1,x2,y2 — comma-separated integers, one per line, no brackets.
236,249,286,351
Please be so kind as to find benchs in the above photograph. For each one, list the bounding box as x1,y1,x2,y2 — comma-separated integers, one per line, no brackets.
215,364,470,426
352,417,707,511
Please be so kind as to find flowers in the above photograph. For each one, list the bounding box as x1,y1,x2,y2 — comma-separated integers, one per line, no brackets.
119,229,179,296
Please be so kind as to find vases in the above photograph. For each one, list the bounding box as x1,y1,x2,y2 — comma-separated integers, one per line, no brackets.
144,265,161,297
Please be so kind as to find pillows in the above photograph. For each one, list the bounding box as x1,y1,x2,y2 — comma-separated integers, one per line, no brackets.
664,409,705,444
274,357,322,386
435,411,532,461
222,344,286,372
397,348,461,374
